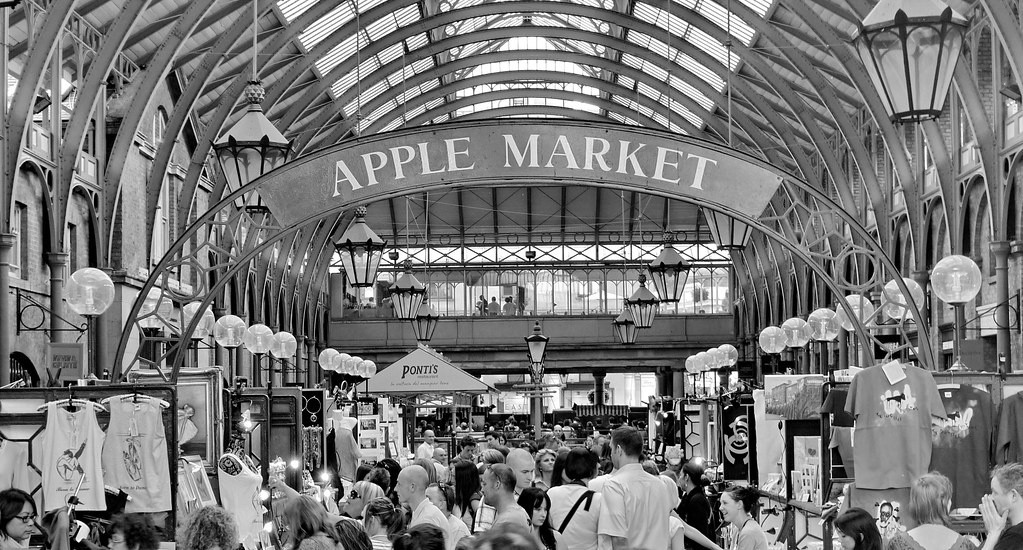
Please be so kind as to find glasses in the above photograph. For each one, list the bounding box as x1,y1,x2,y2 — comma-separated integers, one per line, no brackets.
436,480,448,502
15,515,37,523
111,535,125,543
486,463,501,482
351,490,361,499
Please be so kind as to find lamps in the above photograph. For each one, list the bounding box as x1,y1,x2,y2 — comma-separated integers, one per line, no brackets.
526,250,537,259
612,192,639,345
33,95,52,114
387,41,427,320
387,252,399,260
208,0,295,217
701,0,752,251
332,0,388,288
647,0,692,302
685,254,1021,381
999,83,1022,101
624,80,660,329
410,189,439,342
16,268,376,379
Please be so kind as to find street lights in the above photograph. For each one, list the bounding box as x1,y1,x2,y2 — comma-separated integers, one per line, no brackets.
213,314,248,387
318,347,377,405
931,253,984,370
133,289,177,367
879,277,922,369
510,317,562,442
269,330,297,388
244,322,275,386
685,296,875,395
62,265,117,381
175,300,216,365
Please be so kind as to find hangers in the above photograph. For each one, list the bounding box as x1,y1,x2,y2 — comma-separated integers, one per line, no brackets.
884,347,905,371
37,383,110,413
936,370,961,390
100,379,171,408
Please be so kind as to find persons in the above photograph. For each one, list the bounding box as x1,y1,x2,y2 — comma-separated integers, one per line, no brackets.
277,429,568,550
422,414,769,550
475,295,516,316
0,487,239,550
366,297,376,308
835,462,1023,550
